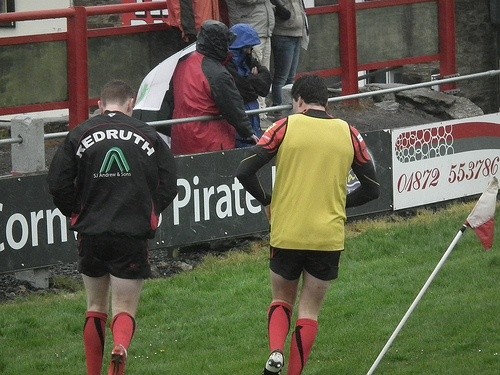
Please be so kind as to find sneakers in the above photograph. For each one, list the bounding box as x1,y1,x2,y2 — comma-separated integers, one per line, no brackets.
108,345,128,375
262,348,286,375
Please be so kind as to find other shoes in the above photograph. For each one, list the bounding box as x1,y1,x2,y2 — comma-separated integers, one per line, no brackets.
272,115,286,124
173,253,196,271
260,118,273,132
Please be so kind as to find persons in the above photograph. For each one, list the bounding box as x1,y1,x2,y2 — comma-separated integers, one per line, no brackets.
225,0,275,118
229,22,271,147
48,80,176,375
171,20,259,155
167,0,221,46
271,0,309,116
237,74,380,374
